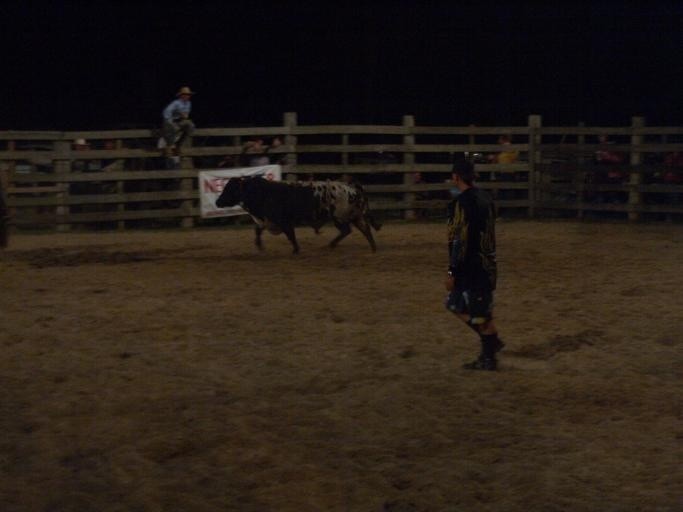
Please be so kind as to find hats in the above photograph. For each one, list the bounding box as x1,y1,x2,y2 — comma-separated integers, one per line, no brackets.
177,87,194,96
73,139,88,145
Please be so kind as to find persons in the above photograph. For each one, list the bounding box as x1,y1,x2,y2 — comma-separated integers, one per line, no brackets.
217,136,288,181
449,124,482,165
67,138,125,230
161,86,197,156
654,151,683,224
444,157,504,372
487,135,521,223
590,132,626,221
413,171,433,218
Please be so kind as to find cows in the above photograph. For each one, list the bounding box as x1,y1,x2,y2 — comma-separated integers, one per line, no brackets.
215,172,383,255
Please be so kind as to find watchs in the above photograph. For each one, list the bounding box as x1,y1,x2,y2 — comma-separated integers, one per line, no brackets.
447,272,458,278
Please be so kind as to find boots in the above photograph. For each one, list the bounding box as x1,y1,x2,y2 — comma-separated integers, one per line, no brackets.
463,334,504,370
